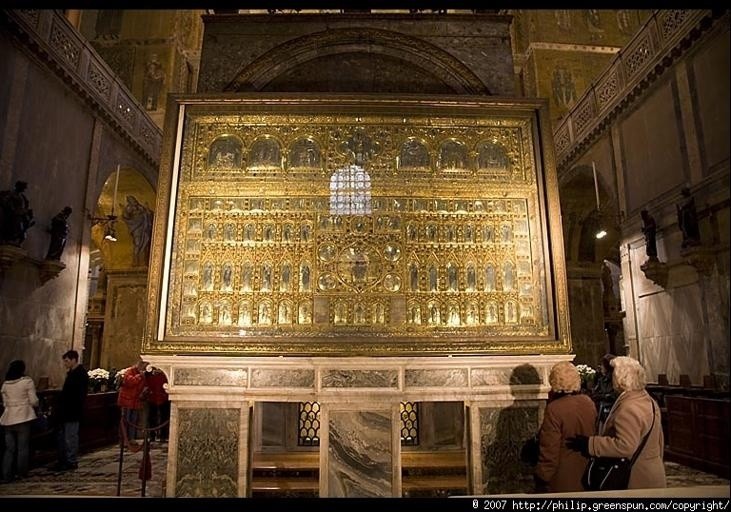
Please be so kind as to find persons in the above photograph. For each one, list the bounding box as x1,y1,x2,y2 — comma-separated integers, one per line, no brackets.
0,359,40,481
638,210,658,262
142,364,170,443
602,262,619,317
55,348,90,471
0,179,39,247
122,194,154,267
46,204,74,261
115,362,153,443
564,356,667,490
672,187,702,249
586,353,617,427
521,360,598,492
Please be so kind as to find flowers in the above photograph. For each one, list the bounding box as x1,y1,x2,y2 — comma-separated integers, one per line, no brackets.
87,368,110,382
576,364,596,388
114,367,132,384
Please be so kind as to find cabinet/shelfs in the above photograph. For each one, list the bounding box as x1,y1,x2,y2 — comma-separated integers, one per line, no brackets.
645,385,729,480
29,389,118,471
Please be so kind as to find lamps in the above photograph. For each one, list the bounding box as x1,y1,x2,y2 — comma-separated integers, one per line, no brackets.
592,160,607,239
104,164,120,242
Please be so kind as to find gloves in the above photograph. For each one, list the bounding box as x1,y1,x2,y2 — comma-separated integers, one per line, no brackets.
566,434,589,453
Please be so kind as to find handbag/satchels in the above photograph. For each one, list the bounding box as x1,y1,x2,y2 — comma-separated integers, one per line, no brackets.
582,456,631,490
